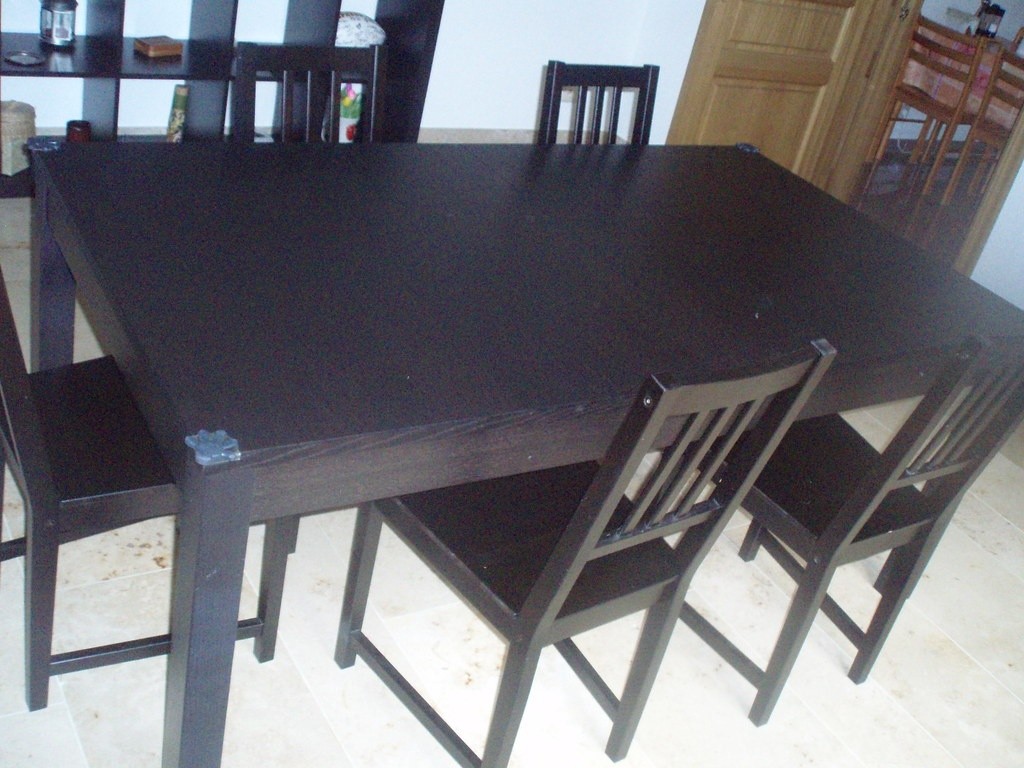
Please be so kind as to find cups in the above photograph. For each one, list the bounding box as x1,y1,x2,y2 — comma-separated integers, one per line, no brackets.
38,0,79,50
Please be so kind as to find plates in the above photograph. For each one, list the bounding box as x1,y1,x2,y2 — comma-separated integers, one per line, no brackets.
4,51,47,67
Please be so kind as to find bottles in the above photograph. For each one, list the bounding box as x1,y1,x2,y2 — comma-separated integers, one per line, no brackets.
974,0,1006,39
67,120,91,143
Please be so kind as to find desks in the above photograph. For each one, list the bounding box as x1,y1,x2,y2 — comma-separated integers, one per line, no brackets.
900,24,1024,132
26,138,1024,768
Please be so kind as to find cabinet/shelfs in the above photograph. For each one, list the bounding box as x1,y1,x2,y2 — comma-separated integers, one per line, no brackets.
0,0,444,200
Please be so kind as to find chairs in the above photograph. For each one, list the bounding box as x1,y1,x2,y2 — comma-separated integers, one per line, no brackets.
675,332,1022,727
875,17,1024,221
230,40,382,552
334,341,837,768
540,59,659,144
1,271,289,712
